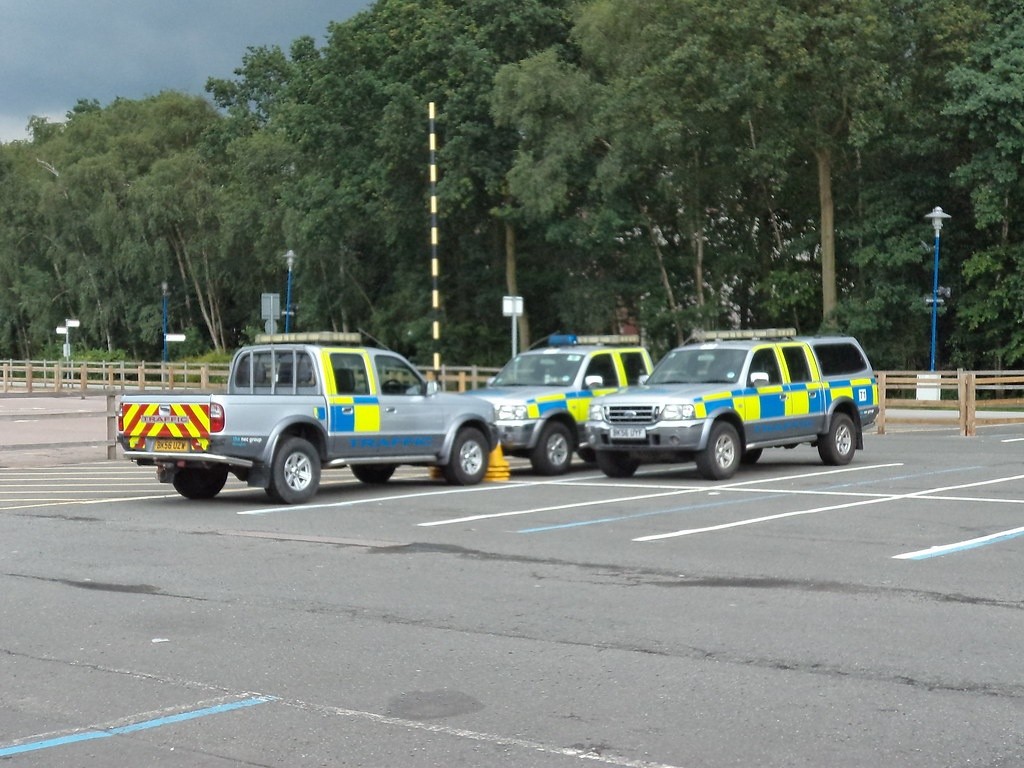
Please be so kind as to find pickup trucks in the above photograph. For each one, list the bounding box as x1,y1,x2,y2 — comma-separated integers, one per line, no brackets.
461,331,681,476
115,341,501,505
583,328,883,483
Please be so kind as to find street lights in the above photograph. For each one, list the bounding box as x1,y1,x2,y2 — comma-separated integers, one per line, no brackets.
160,277,170,389
284,248,298,332
926,207,952,372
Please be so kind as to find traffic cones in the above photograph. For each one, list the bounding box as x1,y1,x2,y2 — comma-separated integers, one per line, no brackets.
484,437,512,484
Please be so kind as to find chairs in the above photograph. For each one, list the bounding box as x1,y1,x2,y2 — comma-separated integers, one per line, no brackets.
291,354,312,384
334,369,355,394
246,361,266,385
